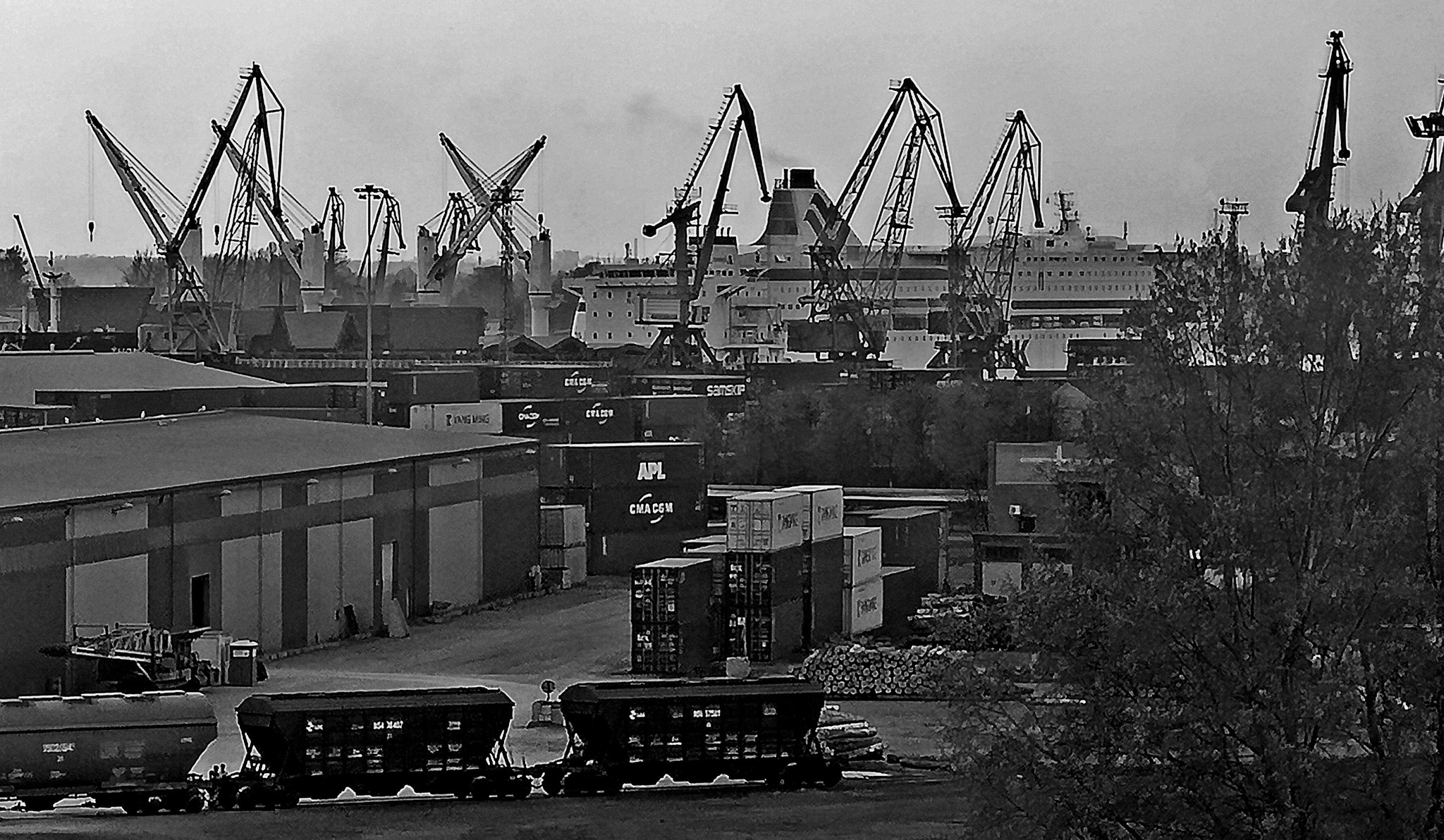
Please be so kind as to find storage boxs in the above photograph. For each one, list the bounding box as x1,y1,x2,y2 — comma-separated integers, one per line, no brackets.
378,359,947,670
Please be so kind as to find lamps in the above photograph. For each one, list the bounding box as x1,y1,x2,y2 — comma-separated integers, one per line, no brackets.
514,449,537,457
381,467,397,477
2,517,23,529
452,458,469,468
303,478,319,489
111,502,133,519
213,487,232,498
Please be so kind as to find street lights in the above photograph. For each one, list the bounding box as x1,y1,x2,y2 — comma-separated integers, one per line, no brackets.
356,193,382,427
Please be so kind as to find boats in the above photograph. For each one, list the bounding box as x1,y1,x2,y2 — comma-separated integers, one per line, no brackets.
554,168,1209,382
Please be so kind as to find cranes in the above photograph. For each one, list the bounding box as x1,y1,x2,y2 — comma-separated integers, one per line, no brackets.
780,76,970,381
1283,30,1353,269
85,61,552,368
633,82,774,378
920,107,1045,383
